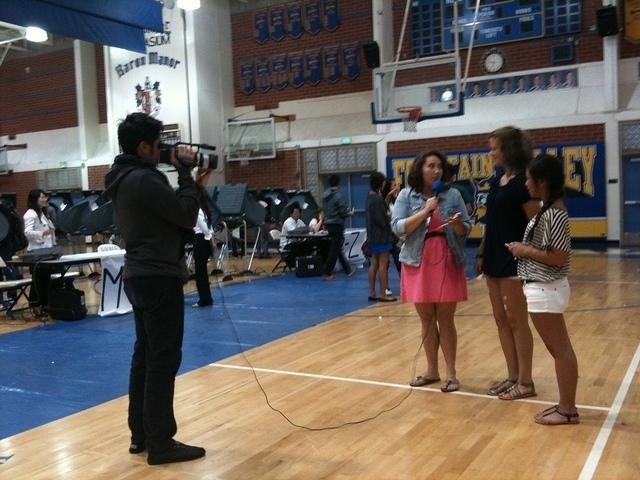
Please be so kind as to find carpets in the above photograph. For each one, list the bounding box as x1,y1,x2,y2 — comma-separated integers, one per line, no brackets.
0,242,480,441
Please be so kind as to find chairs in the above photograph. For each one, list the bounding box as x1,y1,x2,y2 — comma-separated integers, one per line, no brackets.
51,272,80,279
0,256,32,319
97,244,121,252
269,229,293,273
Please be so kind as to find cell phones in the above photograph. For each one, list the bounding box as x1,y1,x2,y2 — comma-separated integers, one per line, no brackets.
504,243,511,247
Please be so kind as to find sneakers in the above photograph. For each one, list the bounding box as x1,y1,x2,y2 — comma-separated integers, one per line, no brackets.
346,267,358,276
129,441,147,454
322,272,334,280
148,439,206,464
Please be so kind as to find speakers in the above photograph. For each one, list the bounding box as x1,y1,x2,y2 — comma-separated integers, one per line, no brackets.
363,41,379,69
596,6,618,37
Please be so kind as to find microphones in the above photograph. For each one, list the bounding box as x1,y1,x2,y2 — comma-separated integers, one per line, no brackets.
424,179,444,230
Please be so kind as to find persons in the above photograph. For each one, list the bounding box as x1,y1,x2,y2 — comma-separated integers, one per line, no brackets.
476,126,542,401
322,175,356,281
361,172,397,301
1,197,30,311
391,150,471,392
194,185,215,308
103,112,212,466
279,207,306,268
24,189,55,321
309,208,324,233
508,154,580,425
382,178,401,295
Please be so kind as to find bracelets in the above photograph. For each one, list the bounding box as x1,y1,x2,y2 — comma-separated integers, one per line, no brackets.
475,253,484,258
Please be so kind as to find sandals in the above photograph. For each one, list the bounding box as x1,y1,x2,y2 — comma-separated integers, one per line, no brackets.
536,410,581,425
379,294,396,301
499,382,538,400
488,379,517,395
367,295,379,300
412,374,439,387
442,376,461,393
534,406,559,421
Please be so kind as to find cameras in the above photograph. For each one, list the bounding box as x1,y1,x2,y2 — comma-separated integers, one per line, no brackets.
157,141,218,171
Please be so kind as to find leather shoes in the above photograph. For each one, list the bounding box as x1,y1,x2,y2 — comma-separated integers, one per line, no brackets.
192,301,207,307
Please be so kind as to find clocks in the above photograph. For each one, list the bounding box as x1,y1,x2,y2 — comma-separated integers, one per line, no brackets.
480,47,507,75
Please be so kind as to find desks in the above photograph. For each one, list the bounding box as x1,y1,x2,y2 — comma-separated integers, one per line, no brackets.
286,228,368,269
6,250,133,317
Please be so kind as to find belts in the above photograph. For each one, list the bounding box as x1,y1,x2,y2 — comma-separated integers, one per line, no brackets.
427,231,447,238
